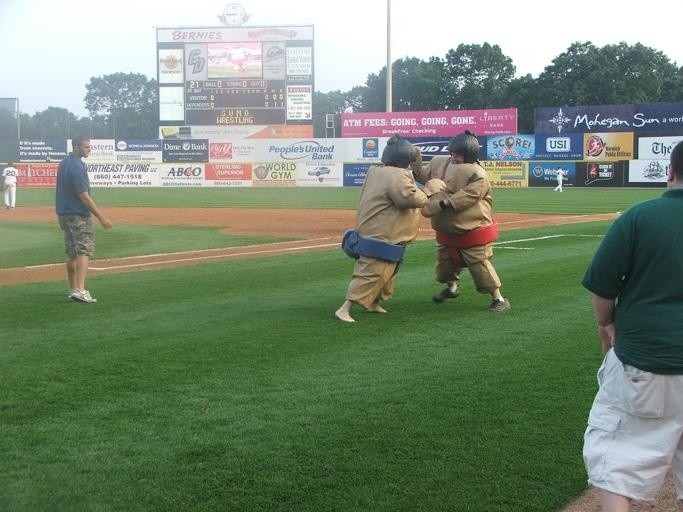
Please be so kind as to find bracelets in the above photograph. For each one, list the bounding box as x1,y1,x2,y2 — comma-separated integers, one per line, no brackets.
597,317,614,326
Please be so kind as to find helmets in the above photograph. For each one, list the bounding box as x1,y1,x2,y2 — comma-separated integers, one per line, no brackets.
381,134,415,164
449,130,480,164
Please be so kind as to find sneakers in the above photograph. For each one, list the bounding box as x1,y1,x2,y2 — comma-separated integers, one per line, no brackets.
68,289,97,303
433,284,459,303
487,297,511,311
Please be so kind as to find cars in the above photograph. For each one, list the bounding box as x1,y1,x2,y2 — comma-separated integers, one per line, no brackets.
308,167,330,176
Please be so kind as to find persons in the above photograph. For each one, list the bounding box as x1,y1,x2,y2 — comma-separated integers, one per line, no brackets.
408,128,511,313
553,167,564,192
1,161,19,209
55,132,113,304
332,132,448,324
581,141,682,512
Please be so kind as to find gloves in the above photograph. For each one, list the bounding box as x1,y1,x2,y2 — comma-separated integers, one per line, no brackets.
421,201,443,218
410,147,423,175
422,178,446,198
